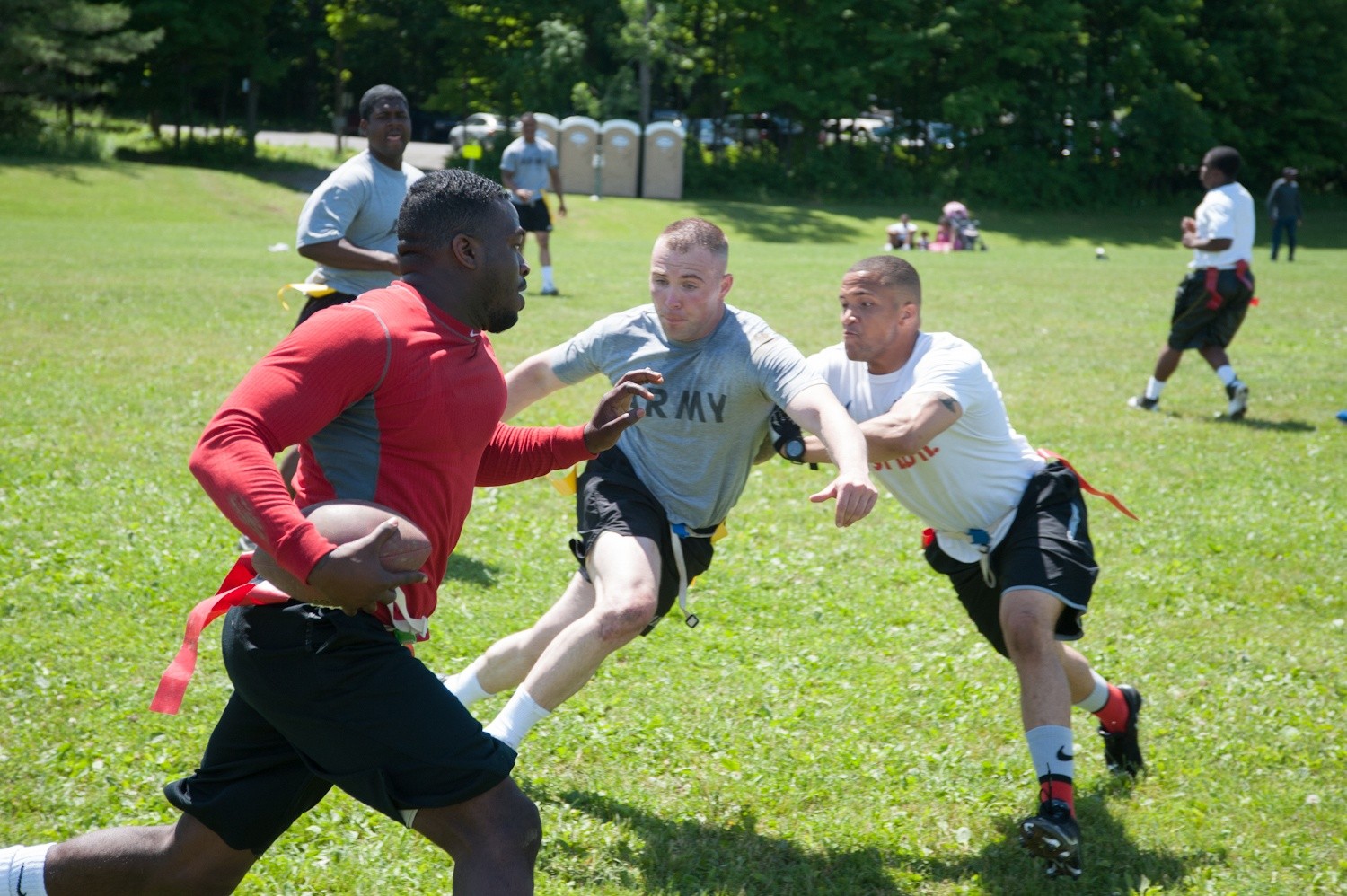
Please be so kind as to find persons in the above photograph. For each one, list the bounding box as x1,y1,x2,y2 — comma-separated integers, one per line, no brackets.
238,84,430,554
752,255,1145,879
0,169,664,896
1266,167,1303,262
499,113,567,296
1128,147,1256,420
426,219,879,753
883,212,955,252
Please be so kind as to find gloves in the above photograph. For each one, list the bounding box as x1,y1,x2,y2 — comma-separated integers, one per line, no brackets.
764,405,808,467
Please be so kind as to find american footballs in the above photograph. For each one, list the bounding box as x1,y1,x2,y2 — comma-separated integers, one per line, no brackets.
247,496,434,608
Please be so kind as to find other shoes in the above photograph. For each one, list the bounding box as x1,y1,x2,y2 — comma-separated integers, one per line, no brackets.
541,287,558,295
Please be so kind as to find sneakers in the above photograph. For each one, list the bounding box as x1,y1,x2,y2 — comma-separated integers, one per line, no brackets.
1130,392,1162,414
1096,685,1143,777
1020,762,1084,880
1227,379,1250,420
